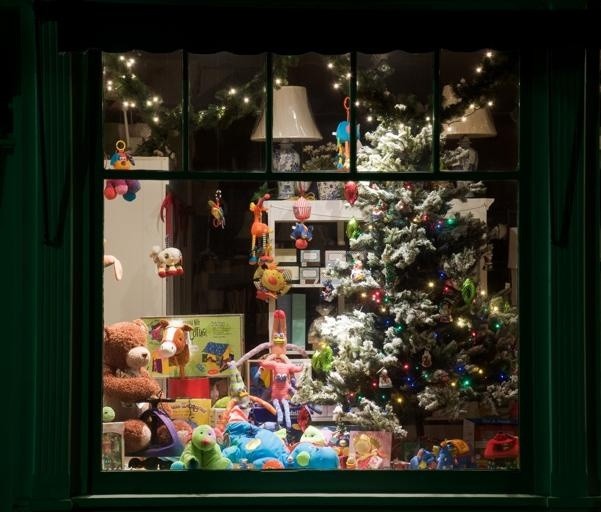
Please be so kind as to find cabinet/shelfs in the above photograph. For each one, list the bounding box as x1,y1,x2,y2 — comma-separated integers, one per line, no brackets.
102,156,176,402
263,196,493,367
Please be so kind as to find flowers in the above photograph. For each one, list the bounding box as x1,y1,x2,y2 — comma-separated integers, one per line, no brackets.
303,142,343,171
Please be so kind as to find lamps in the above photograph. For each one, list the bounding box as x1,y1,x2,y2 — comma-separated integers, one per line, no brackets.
438,89,499,194
250,82,321,198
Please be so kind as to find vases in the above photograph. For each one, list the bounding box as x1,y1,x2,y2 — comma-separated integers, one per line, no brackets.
316,173,344,198
